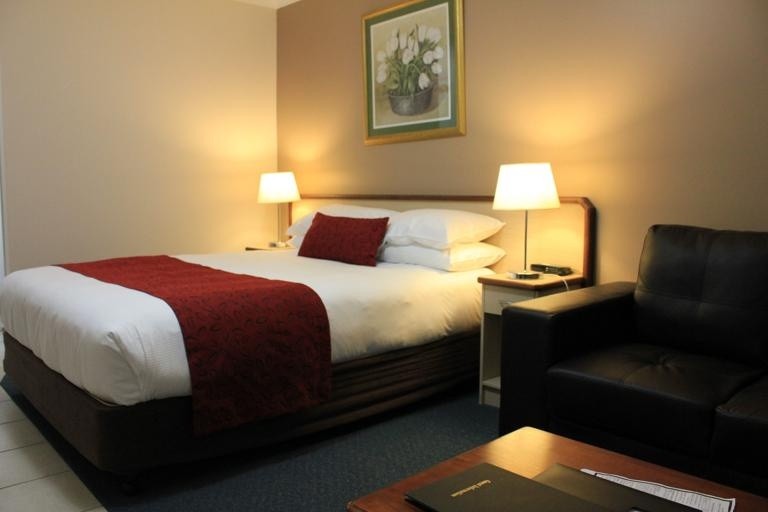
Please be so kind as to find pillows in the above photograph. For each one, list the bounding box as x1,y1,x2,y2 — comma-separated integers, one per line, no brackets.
284,204,507,273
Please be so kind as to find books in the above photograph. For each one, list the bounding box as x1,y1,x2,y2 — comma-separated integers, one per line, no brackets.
403,462,704,511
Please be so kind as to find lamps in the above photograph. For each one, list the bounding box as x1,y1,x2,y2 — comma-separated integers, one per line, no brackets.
491,161,562,280
256,171,302,248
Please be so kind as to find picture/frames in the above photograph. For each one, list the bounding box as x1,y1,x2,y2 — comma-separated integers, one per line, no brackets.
360,0,468,147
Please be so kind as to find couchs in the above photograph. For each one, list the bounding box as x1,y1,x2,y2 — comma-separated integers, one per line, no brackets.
498,224,767,497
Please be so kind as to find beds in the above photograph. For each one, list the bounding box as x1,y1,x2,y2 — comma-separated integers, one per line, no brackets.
0,193,596,497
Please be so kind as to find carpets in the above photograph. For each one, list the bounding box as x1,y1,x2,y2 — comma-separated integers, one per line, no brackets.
0,373,509,512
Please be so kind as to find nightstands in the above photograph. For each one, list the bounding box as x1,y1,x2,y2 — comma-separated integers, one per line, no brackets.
476,273,587,409
246,246,295,251
